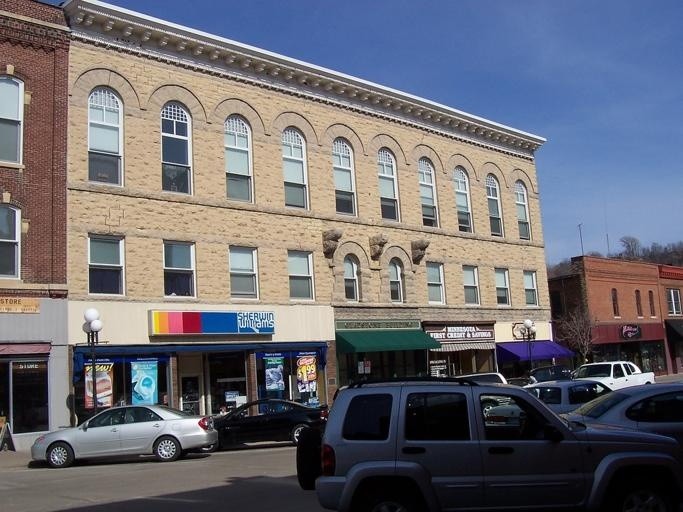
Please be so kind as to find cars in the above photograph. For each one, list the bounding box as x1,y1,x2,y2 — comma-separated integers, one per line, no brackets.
556,380,683,441
453,365,573,422
487,380,613,425
571,360,656,391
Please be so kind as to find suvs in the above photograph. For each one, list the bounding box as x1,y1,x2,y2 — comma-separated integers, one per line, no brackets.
197,398,330,453
31,404,218,469
296,375,682,512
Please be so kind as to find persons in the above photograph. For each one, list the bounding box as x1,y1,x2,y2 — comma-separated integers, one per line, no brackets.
265,364,283,387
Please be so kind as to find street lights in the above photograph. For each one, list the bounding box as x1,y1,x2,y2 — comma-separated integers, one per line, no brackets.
82,308,103,417
520,319,536,371
